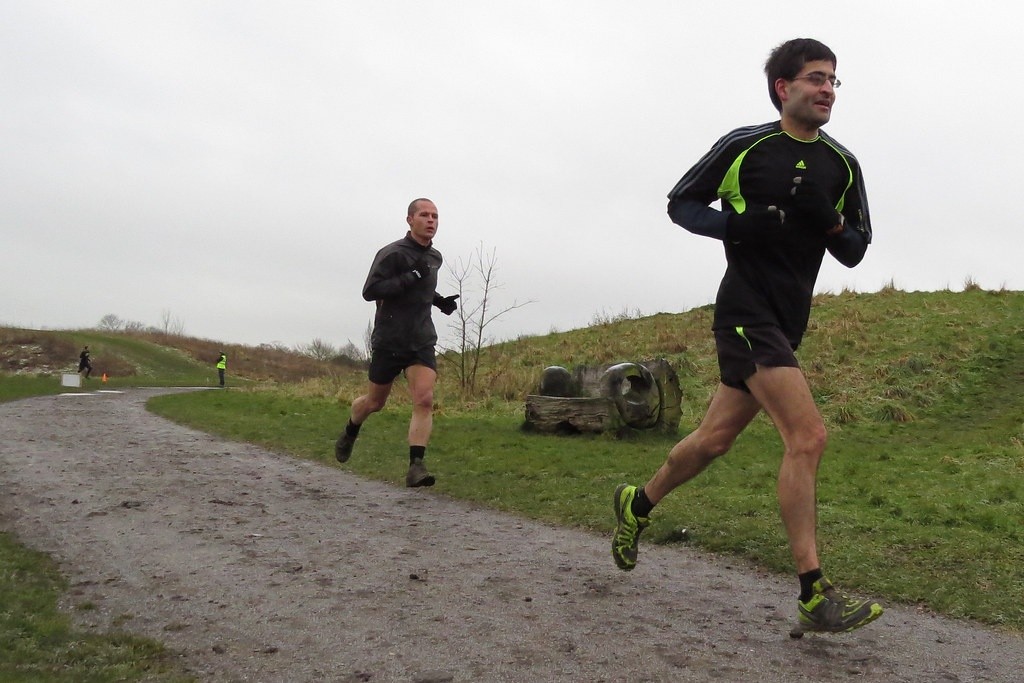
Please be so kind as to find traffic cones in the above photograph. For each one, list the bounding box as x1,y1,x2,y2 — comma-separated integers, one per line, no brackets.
101,373,108,382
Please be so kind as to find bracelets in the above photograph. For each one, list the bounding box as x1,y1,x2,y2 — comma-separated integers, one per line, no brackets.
832,211,844,234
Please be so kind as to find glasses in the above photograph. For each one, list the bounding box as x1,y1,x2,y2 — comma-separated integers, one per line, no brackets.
782,73,841,88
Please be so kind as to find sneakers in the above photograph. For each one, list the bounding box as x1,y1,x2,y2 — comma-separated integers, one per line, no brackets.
797,576,885,633
336,422,357,463
405,457,435,489
611,483,652,572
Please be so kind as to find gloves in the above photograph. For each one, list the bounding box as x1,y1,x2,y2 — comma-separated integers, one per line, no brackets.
414,258,430,278
439,294,461,316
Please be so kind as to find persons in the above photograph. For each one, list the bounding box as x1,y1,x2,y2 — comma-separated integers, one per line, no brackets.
610,39,883,633
216,350,227,388
334,199,460,488
77,346,92,379
